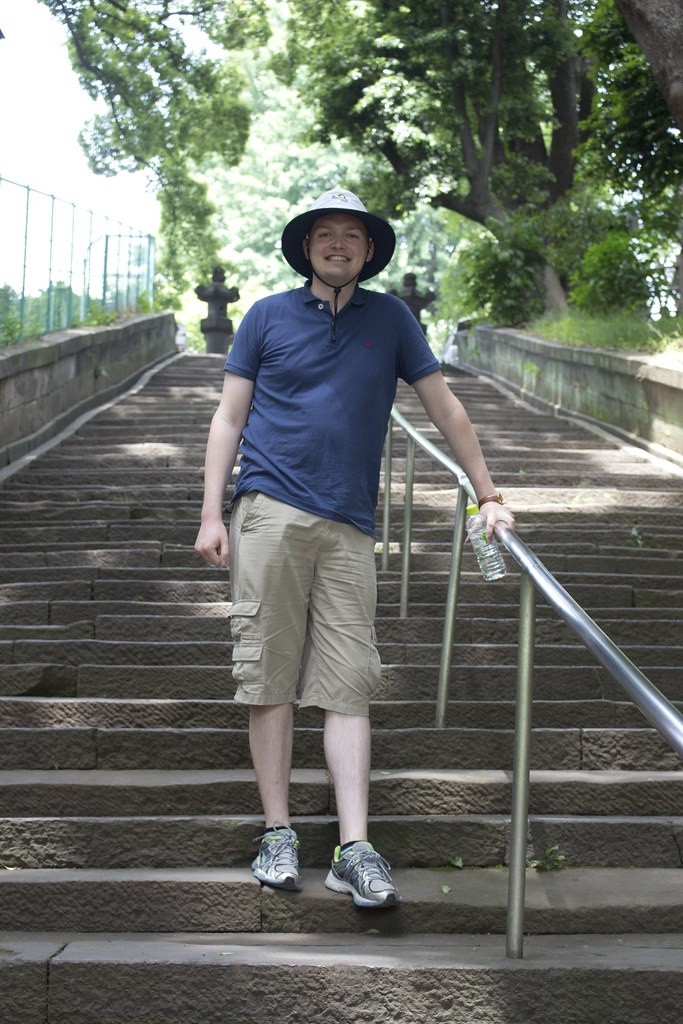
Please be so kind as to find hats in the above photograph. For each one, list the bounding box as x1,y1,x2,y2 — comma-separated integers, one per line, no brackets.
281,189,396,284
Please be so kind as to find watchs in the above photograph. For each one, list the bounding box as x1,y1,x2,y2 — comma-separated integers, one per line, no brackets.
478,494,504,511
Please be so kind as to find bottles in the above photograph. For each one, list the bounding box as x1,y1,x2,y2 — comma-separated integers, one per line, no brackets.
465,505,508,581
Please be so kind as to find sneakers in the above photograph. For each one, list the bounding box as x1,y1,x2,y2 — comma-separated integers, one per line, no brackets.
325,842,403,908
251,829,304,891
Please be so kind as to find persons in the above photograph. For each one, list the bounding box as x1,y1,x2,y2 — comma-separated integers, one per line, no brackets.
195,186,516,908
